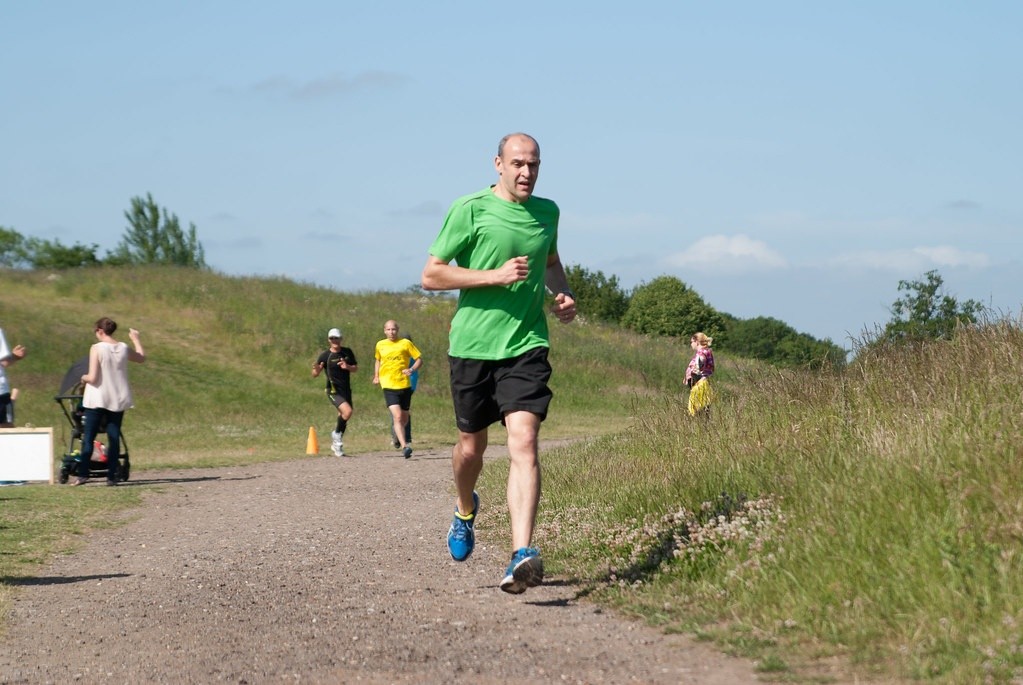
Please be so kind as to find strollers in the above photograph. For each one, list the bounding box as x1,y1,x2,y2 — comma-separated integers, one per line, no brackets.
54,359,131,485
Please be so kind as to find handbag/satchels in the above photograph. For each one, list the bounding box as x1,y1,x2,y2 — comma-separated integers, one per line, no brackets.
689,371,713,417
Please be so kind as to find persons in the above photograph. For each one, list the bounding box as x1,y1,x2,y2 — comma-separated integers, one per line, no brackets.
683,332,715,419
421,133,578,595
68,317,146,486
373,319,423,458
0,328,26,428
390,331,419,449
312,328,358,457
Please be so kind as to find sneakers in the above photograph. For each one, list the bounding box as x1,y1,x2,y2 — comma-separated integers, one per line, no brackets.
331,444,344,457
332,430,343,451
447,490,480,562
500,548,543,594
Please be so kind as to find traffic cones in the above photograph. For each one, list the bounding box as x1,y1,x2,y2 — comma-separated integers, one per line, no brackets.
306,426,320,456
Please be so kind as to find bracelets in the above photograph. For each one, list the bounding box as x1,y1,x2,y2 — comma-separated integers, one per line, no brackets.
560,291,574,299
411,367,414,371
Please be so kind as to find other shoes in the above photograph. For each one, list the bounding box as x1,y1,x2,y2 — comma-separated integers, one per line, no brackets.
402,447,412,459
0,480,27,486
107,479,117,485
70,476,88,486
391,431,400,448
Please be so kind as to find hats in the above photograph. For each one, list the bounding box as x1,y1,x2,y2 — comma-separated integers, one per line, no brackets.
328,328,341,338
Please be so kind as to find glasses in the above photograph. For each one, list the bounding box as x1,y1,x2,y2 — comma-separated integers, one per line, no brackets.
329,337,338,340
